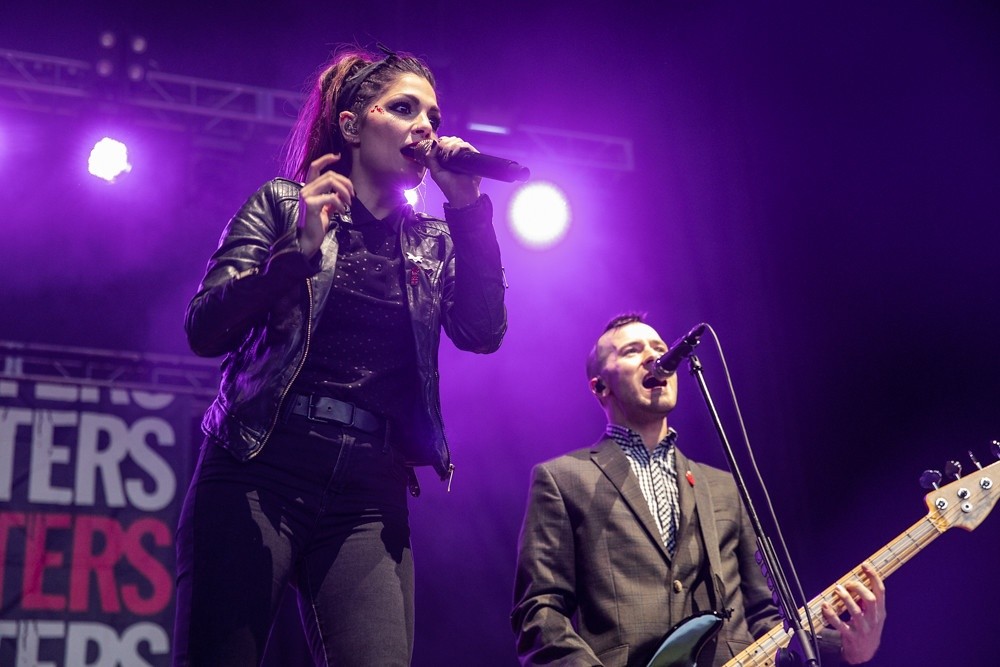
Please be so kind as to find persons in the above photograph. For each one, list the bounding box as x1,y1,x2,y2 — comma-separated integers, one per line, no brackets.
167,49,511,667
513,310,886,666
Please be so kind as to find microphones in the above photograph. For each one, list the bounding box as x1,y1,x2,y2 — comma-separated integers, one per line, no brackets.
651,322,707,381
414,138,530,183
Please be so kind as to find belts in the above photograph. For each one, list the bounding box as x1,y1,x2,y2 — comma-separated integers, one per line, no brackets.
286,396,407,442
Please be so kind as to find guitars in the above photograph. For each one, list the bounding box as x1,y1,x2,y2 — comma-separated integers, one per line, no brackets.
646,439,1000,667
